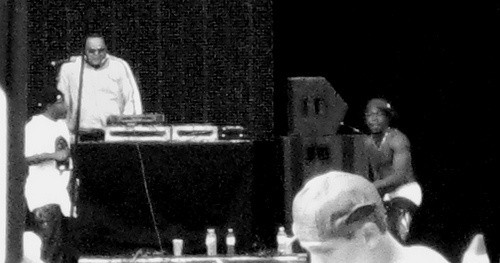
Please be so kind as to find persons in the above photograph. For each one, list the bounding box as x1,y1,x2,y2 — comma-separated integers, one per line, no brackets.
364,98,423,245
56,32,143,217
293,171,452,263
24,89,84,263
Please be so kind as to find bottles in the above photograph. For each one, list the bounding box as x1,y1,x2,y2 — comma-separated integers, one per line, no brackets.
205,228,217,256
277,226,288,256
225,228,236,256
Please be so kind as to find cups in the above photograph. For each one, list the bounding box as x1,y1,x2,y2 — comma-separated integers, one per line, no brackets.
172,239,183,256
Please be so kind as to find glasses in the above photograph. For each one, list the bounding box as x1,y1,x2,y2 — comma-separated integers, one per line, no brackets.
86,45,107,54
364,109,387,116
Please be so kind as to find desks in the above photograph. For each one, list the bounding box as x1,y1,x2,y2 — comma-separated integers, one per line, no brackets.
70,141,274,254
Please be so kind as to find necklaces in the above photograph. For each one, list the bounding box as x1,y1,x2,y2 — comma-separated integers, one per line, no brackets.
371,127,390,151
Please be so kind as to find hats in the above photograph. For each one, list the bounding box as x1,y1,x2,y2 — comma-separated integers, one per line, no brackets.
291,170,386,242
39,89,63,109
362,98,392,109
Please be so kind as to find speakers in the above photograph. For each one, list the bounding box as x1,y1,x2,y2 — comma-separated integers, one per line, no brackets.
287,76,337,136
280,134,374,254
